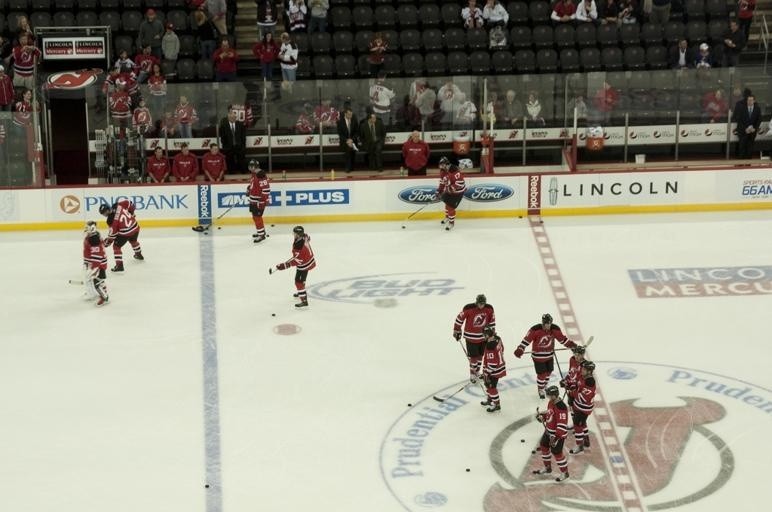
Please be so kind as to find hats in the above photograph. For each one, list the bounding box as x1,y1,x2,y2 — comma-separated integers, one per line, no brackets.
146,9,155,16
700,43,709,50
166,23,173,28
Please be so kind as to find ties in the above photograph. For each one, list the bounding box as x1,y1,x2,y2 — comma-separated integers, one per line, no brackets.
749,107,752,118
371,124,375,141
348,120,351,136
231,124,236,147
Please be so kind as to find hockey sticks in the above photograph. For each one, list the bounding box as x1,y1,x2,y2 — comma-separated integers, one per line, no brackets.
553,351,567,401
432,373,485,402
191,195,247,232
458,339,487,397
402,198,438,229
521,335,593,355
269,253,297,275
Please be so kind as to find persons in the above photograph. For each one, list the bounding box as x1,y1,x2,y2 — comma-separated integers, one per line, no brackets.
437,160,468,231
479,328,508,413
316,98,335,136
415,81,437,132
11,38,42,105
337,103,352,132
160,112,177,138
115,51,135,72
456,93,477,128
100,198,144,273
564,362,599,454
17,16,32,47
454,294,496,387
173,143,200,185
0,65,15,112
199,0,227,37
619,0,636,24
136,46,159,83
359,112,388,170
669,40,696,90
336,110,363,173
147,64,167,96
737,0,756,40
253,1,277,43
145,146,171,185
598,1,620,25
486,27,508,47
310,0,329,35
551,1,577,21
279,33,298,82
222,106,248,177
161,23,180,76
369,70,396,130
368,31,388,78
211,39,239,81
483,0,510,27
177,96,196,134
138,8,164,53
437,76,467,113
576,1,598,21
297,103,319,134
193,12,216,62
396,96,423,133
461,0,485,30
17,92,36,161
402,130,432,176
561,349,588,429
360,106,381,127
132,100,152,133
103,68,138,133
695,41,714,90
703,87,730,122
500,89,524,128
567,96,587,127
276,224,315,308
533,385,572,483
202,146,227,185
513,312,588,399
597,81,618,123
724,21,747,68
731,87,751,123
526,92,545,130
738,96,762,166
80,221,108,309
253,33,279,82
286,1,308,37
244,161,271,243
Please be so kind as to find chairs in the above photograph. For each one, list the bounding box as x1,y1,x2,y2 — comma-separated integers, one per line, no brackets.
0,0,236,186
280,0,738,134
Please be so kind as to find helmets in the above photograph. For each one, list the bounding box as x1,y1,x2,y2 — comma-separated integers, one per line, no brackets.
543,314,553,325
439,156,451,165
546,386,559,395
581,361,595,370
573,346,585,354
84,221,97,236
100,204,110,214
139,97,145,101
327,105,336,113
416,82,426,91
294,226,304,233
304,103,313,116
375,78,385,86
476,295,487,303
483,326,495,333
248,160,259,166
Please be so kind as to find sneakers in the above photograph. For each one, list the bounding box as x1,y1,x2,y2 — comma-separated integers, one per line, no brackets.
134,254,144,260
252,233,259,237
470,377,477,383
480,400,492,405
111,265,123,272
539,467,552,474
97,297,109,304
545,386,549,391
294,293,299,297
476,374,481,378
583,441,590,447
446,221,454,229
254,234,265,242
538,389,545,397
442,218,449,224
295,301,308,307
486,405,500,412
556,472,569,481
570,445,584,453
